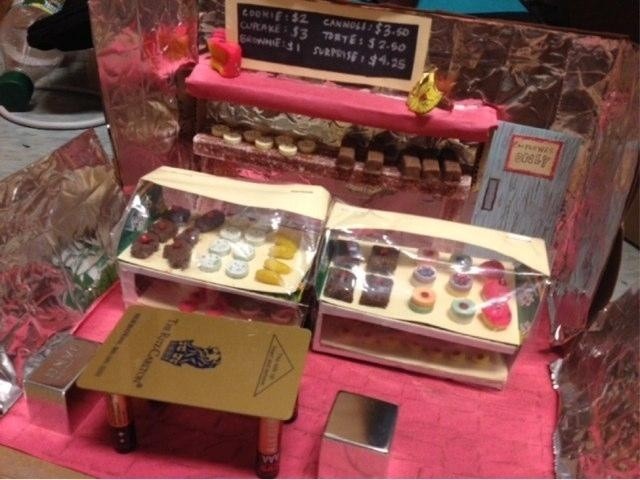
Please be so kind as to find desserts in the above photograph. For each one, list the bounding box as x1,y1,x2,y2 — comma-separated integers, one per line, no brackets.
131,204,301,289
324,241,514,334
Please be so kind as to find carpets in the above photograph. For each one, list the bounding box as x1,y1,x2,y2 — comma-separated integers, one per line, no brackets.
0,278,558,479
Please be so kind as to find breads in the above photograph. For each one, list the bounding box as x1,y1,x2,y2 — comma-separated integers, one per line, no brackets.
211,122,462,180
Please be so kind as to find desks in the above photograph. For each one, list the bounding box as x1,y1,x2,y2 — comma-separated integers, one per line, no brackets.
115,209,320,327
309,235,520,392
75,306,312,479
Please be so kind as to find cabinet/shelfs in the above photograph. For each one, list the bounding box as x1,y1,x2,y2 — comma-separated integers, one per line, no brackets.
185,61,498,221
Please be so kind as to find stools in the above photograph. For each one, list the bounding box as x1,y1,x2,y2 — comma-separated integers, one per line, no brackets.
23,334,104,436
317,389,398,479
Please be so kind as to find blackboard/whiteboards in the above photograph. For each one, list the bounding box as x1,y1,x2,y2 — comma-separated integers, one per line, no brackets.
224,0,432,93
